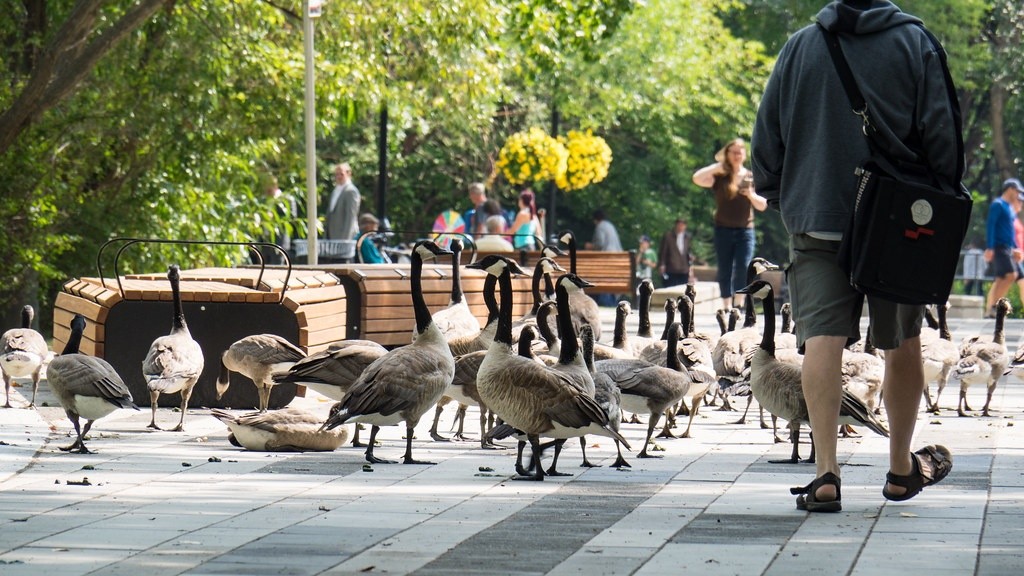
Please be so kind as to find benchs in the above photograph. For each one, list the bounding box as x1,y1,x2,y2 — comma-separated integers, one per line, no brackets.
52,234,654,406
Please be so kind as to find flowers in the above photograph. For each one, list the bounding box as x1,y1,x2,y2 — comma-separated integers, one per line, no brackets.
496,126,612,191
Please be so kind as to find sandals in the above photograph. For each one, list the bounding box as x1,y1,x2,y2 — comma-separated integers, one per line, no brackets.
790,471,842,513
882,444,953,501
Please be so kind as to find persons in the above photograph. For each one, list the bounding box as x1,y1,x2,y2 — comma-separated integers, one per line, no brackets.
325,163,361,263
464,182,512,243
658,216,695,286
962,240,989,295
635,235,657,283
692,137,768,309
752,0,966,513
355,214,383,264
585,211,623,307
983,181,1024,319
264,177,296,220
505,193,543,250
1013,194,1024,304
476,216,514,252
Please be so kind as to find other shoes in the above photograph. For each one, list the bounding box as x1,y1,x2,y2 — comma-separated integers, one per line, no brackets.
984,310,996,319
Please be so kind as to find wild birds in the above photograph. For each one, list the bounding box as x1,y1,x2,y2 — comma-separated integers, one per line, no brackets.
288,340,390,446
1003,334,1024,381
216,334,306,413
919,304,940,340
202,405,349,453
772,303,886,443
955,298,1013,417
919,301,960,413
47,313,140,454
0,305,49,410
363,239,455,465
447,230,762,481
411,239,480,442
736,280,890,464
142,264,204,433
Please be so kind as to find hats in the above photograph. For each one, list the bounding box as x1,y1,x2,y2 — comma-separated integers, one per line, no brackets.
1017,192,1024,201
1001,178,1024,193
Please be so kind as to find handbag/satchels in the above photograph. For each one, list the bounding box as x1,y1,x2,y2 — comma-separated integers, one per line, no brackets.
849,166,973,307
